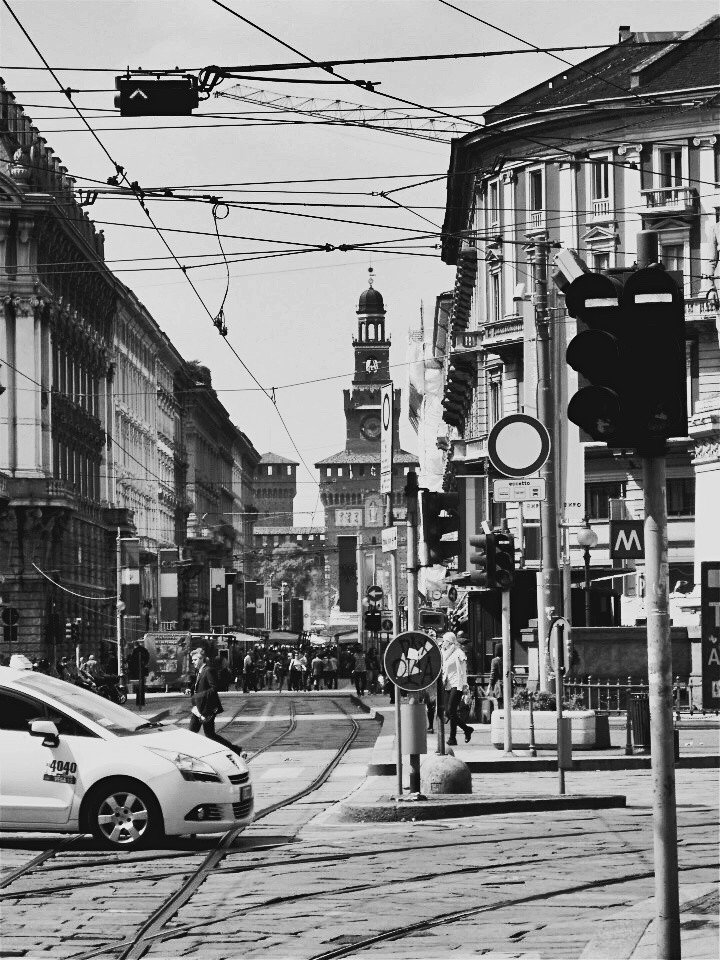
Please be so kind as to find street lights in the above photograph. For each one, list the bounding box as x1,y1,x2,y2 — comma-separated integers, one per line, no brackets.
577,527,599,620
116,601,128,692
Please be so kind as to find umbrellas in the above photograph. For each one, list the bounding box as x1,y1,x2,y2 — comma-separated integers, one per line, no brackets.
218,631,263,641
262,631,304,639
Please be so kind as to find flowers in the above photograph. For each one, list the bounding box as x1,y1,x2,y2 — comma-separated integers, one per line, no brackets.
506,690,595,710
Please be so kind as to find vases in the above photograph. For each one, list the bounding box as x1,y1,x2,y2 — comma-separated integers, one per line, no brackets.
490,709,598,751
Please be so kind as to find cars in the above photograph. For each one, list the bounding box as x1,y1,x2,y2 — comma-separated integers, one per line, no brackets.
0,657,254,849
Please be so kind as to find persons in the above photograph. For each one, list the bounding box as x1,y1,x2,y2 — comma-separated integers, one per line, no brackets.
185,648,242,757
33,654,102,693
440,632,474,745
485,642,503,707
236,643,408,705
423,690,437,733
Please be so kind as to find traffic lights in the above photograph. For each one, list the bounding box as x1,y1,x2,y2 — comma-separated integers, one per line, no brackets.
465,527,495,590
560,265,691,442
496,526,517,590
423,488,460,554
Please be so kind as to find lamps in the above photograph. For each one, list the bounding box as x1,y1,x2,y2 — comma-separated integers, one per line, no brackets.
114,73,200,116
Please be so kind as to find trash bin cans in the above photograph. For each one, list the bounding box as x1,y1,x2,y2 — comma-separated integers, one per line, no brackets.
630,692,651,748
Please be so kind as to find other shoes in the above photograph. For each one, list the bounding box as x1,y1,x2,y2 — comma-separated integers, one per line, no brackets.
240,750,247,759
447,740,457,745
427,728,433,734
465,727,474,743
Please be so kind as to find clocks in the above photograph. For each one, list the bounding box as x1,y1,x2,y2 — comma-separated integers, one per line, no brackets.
363,418,380,436
